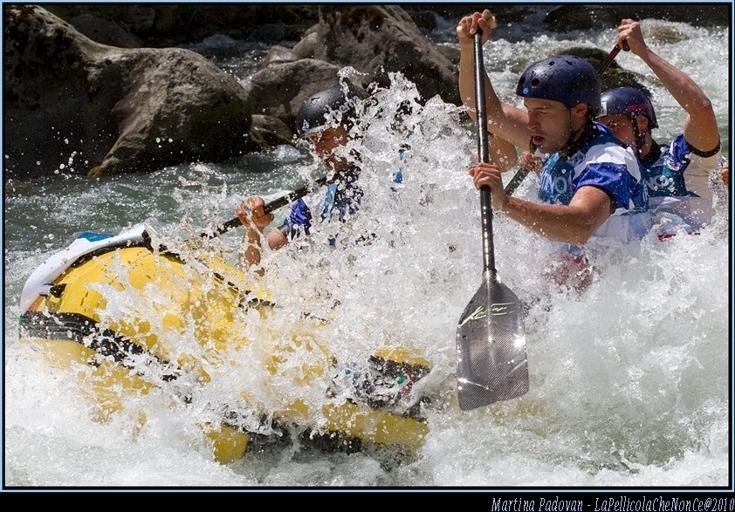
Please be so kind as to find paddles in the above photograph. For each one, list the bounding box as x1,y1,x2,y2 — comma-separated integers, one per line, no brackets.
457,15,530,412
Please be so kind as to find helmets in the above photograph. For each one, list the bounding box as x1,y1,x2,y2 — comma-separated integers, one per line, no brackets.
594,88,659,129
297,87,377,141
516,53,601,116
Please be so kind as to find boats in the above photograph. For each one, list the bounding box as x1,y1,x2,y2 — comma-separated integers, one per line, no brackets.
16,219,553,461
